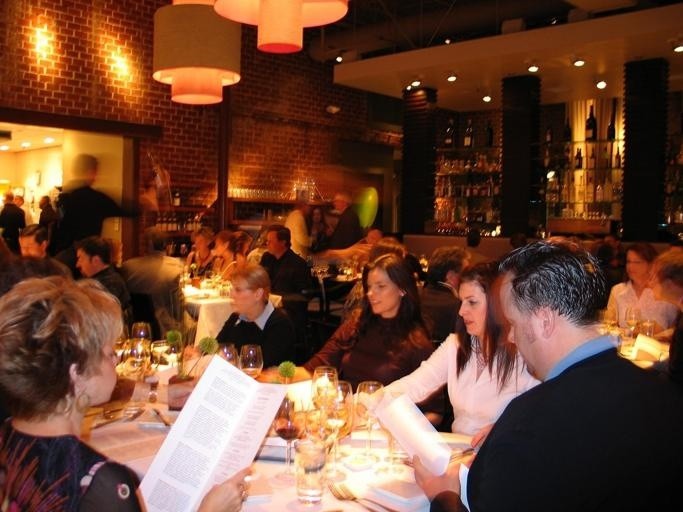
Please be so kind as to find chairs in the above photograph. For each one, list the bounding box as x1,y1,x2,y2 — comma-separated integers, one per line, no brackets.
323,278,355,312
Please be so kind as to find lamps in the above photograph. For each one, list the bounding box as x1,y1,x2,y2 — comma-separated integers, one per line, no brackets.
152,0,241,107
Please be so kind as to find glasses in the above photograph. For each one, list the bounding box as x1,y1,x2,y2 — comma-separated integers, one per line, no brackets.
626,259,647,266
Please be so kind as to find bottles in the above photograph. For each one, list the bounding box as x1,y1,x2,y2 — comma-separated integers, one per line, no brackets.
464,120,474,150
564,119,571,143
441,153,498,175
444,119,455,149
174,189,180,209
589,147,597,167
608,121,614,141
156,212,204,237
604,147,608,166
576,149,582,169
616,147,621,167
586,105,597,141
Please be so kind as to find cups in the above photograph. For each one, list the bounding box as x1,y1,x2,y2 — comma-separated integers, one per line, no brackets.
274,400,307,477
311,367,339,435
303,407,339,466
622,307,641,355
355,382,386,461
240,345,264,382
113,321,174,388
217,342,236,367
182,260,223,298
292,442,327,505
598,308,618,338
321,381,353,481
640,318,659,338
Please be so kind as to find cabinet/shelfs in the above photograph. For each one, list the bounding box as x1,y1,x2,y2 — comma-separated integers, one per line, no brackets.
544,139,624,235
227,197,335,225
435,148,500,236
143,180,216,256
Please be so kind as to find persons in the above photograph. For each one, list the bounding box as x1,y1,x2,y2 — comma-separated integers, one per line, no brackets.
602,243,678,339
414,248,471,344
0,276,253,512
365,228,383,244
411,241,683,512
596,232,628,282
647,247,683,383
465,229,489,261
510,232,527,248
232,232,253,255
0,192,26,250
46,154,136,279
211,231,247,280
0,256,57,294
186,227,216,278
387,232,404,245
74,237,134,326
340,237,407,323
181,263,306,368
260,225,314,330
351,260,543,447
14,196,33,233
122,227,185,317
39,196,55,224
284,199,316,260
307,206,328,249
253,254,445,424
19,224,73,278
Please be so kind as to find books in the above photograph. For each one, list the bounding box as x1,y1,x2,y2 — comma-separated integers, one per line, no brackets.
138,354,288,512
